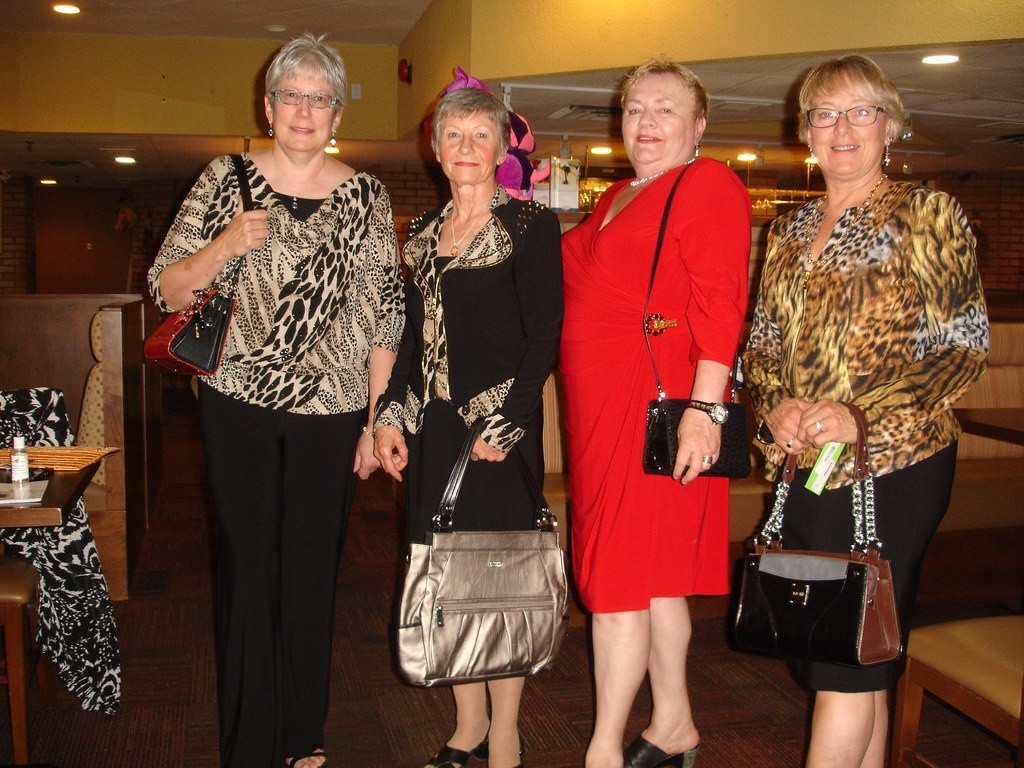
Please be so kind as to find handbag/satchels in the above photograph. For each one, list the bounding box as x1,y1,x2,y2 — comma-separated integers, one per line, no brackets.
721,403,905,668
399,417,568,689
145,154,253,376
644,399,753,478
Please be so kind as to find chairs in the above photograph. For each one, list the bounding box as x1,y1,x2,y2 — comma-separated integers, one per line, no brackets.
891,618,1024,768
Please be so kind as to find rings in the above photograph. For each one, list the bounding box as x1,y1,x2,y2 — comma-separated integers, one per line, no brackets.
786,436,796,448
703,457,713,464
816,421,824,432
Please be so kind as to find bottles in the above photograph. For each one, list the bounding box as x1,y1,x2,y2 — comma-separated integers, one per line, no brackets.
11,437,29,487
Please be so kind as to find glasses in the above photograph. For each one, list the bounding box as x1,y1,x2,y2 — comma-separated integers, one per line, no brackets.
272,89,339,109
807,105,886,128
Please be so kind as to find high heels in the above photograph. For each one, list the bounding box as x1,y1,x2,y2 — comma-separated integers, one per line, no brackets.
424,722,493,768
624,734,701,768
513,733,525,768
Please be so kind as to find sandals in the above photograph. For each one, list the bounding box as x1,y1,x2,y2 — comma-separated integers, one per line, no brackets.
284,748,327,768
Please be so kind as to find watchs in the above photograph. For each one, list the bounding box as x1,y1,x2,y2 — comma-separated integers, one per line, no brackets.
686,400,728,426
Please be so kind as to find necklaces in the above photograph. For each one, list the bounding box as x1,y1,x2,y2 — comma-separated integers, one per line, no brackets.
802,173,886,290
450,197,493,257
630,149,700,187
271,149,327,191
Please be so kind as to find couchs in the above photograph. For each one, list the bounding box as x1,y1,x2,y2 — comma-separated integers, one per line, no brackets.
917,322,1024,609
78,302,142,600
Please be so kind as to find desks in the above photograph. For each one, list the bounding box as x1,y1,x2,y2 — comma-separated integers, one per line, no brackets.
0,459,101,527
953,408,1024,445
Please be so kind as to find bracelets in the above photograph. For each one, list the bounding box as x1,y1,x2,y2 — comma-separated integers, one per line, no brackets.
363,427,374,438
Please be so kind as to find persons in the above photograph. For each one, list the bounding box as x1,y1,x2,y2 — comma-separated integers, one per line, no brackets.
373,65,565,768
740,52,991,768
147,30,406,768
560,53,758,768
